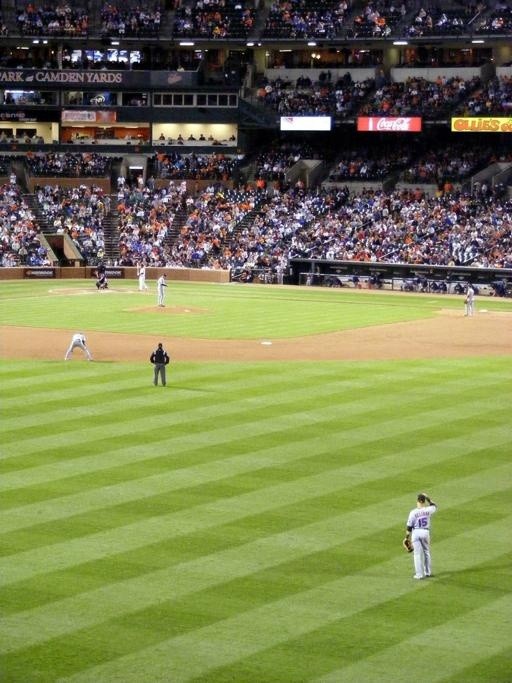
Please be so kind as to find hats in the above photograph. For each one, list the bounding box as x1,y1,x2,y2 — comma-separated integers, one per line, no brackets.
418,494,425,503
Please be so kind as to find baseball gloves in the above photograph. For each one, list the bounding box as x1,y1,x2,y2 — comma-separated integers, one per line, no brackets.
463,299,469,305
94,270,98,274
402,538,414,552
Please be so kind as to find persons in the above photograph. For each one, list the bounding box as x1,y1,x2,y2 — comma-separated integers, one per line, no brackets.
0,1,511,293
65,333,93,360
150,342,170,386
158,273,167,305
403,493,439,578
464,284,474,315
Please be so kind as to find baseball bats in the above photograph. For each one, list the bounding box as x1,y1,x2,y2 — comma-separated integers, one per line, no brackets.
137,262,139,278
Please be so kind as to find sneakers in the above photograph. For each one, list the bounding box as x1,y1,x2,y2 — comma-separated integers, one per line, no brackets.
414,573,430,579
